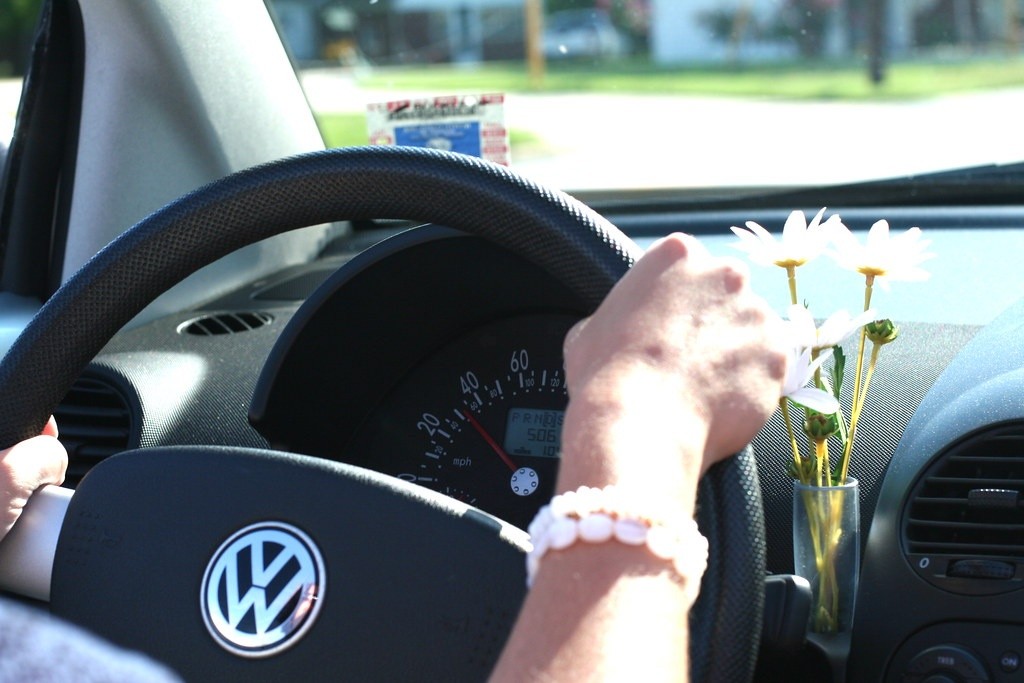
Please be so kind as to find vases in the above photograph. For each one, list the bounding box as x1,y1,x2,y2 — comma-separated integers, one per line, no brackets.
792,476,861,680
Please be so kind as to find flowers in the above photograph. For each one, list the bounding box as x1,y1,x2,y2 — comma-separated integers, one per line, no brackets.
728,206,925,634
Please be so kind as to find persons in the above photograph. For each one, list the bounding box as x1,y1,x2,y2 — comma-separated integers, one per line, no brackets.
0,232,786,683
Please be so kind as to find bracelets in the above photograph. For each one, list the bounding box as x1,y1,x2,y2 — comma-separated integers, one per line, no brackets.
526,483,708,605
